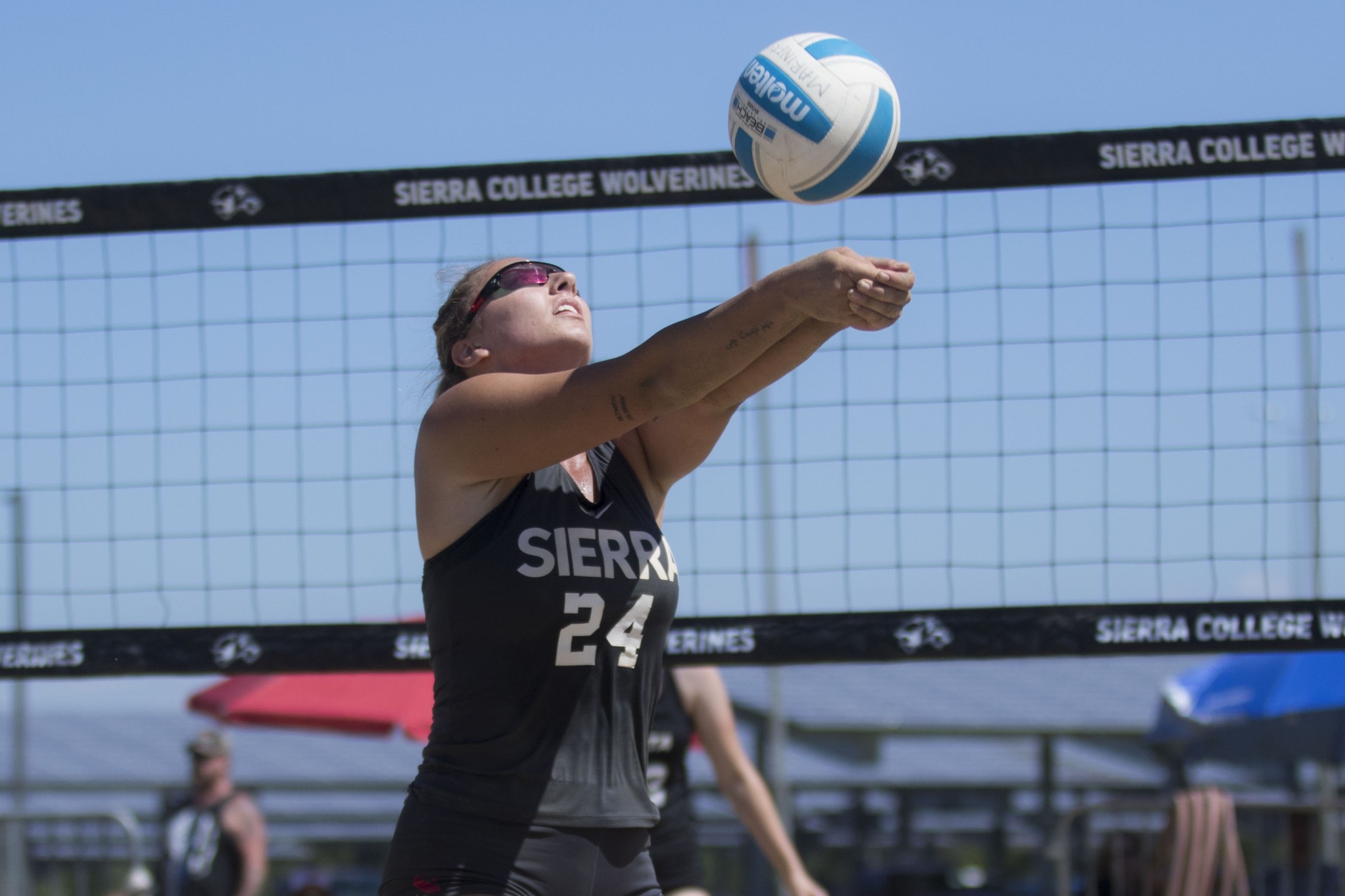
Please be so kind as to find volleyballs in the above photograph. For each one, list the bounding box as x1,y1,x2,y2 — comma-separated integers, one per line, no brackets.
728,32,902,205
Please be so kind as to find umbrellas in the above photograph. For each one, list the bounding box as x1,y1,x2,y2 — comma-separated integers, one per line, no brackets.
186,618,439,743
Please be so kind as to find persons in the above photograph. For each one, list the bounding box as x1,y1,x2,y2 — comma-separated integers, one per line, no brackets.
646,665,828,896
151,729,268,896
374,246,914,896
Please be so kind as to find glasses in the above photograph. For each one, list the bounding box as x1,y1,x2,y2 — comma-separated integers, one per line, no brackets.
452,260,580,339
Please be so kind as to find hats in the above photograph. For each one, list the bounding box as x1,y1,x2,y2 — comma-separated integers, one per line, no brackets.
189,733,227,757
